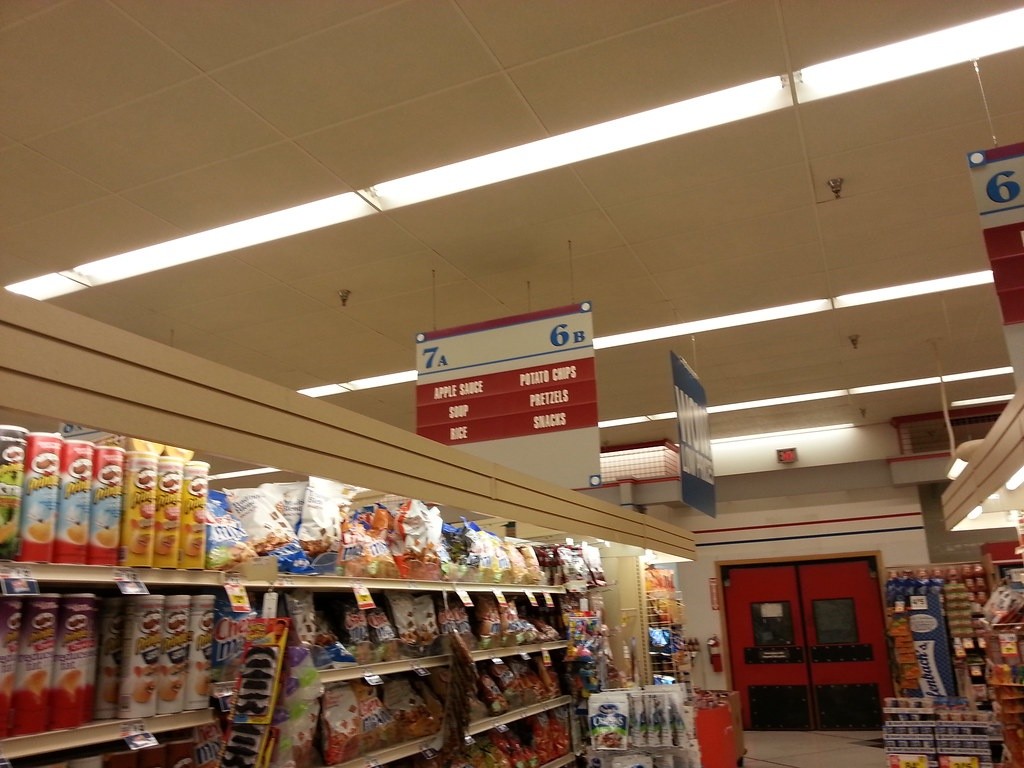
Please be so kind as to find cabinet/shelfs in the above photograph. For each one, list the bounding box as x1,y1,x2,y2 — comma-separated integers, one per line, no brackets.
0,289,700,767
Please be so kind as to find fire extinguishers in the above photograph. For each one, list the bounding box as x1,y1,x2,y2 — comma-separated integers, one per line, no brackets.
706,634,723,672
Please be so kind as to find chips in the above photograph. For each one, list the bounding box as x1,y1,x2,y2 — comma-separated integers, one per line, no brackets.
0,425,216,768
